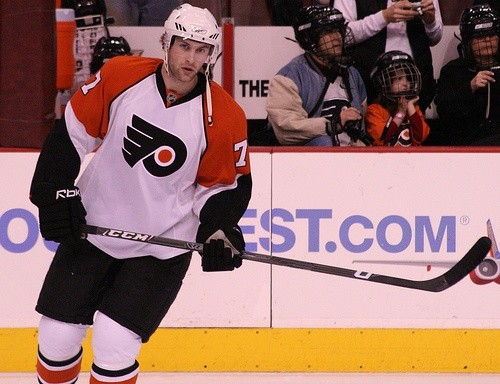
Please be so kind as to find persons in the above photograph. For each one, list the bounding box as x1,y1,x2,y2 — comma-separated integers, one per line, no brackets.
367,52,430,148
89,36,131,76
334,0,444,111
265,5,368,147
30,5,253,384
435,6,500,146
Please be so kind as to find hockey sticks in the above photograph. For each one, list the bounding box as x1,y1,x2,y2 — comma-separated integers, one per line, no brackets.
86,223,493,294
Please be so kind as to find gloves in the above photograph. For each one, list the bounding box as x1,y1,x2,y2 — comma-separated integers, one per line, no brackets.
39,185,89,243
200,237,244,271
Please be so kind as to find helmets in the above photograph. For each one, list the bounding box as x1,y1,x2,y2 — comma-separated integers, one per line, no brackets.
162,3,222,66
293,3,349,63
459,3,500,71
373,50,424,101
93,35,130,64
